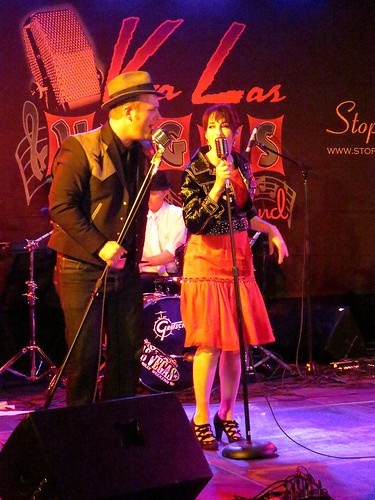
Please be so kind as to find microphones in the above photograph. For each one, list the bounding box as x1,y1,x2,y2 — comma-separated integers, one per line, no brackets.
246,126,258,152
216,138,228,159
152,130,170,148
41,207,49,219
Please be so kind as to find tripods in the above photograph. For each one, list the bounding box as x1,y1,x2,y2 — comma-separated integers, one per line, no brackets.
0,226,63,387
241,345,296,384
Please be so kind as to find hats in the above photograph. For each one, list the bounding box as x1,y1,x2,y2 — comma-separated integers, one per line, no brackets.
100,71,166,110
148,170,173,191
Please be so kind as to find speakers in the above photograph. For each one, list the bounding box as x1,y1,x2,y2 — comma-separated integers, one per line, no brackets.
0,392,214,500
292,305,366,362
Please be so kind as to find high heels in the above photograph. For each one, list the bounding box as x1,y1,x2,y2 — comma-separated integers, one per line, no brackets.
214,411,244,443
191,412,219,450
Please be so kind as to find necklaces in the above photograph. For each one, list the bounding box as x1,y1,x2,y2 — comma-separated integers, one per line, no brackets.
229,161,233,167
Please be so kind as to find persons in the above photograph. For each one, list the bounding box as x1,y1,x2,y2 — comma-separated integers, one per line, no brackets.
48,71,165,408
179,105,288,448
139,171,187,293
